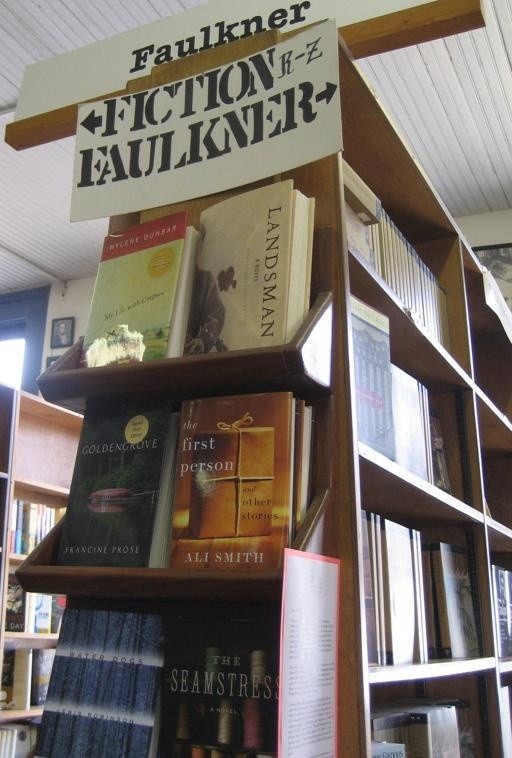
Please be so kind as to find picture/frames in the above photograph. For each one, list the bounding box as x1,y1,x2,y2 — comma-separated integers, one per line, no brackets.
46,356,60,368
50,317,74,347
471,243,511,313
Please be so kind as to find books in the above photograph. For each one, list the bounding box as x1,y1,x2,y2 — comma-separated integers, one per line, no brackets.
340,153,450,354
348,292,471,502
1,648,56,710
7,495,67,555
370,698,474,758
52,393,317,569
80,208,202,366
0,717,42,758
183,178,316,356
360,511,486,669
490,564,512,661
32,604,280,757
6,573,68,634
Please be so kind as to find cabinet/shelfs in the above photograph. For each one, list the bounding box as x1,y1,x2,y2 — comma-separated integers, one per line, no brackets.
0,386,86,758
16,20,512,758
